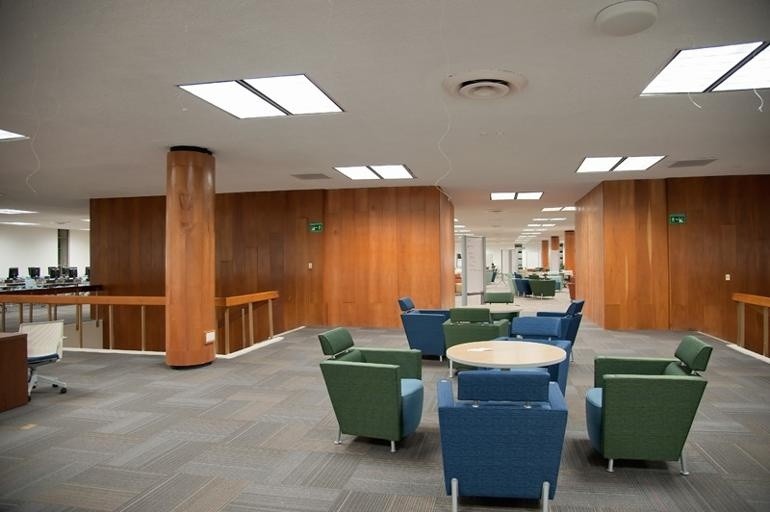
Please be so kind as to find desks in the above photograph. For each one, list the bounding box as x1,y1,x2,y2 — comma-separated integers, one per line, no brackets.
0,332,29,413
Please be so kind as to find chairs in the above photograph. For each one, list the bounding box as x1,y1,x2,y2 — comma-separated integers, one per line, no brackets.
397,291,585,418
436,370,569,512
511,272,561,300
585,335,713,475
20,319,68,401
317,328,424,453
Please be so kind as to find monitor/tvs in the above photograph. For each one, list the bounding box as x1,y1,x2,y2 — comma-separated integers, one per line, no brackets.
28,266,90,282
8,267,19,280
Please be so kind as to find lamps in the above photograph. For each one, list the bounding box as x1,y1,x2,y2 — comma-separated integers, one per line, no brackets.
596,2,659,38
